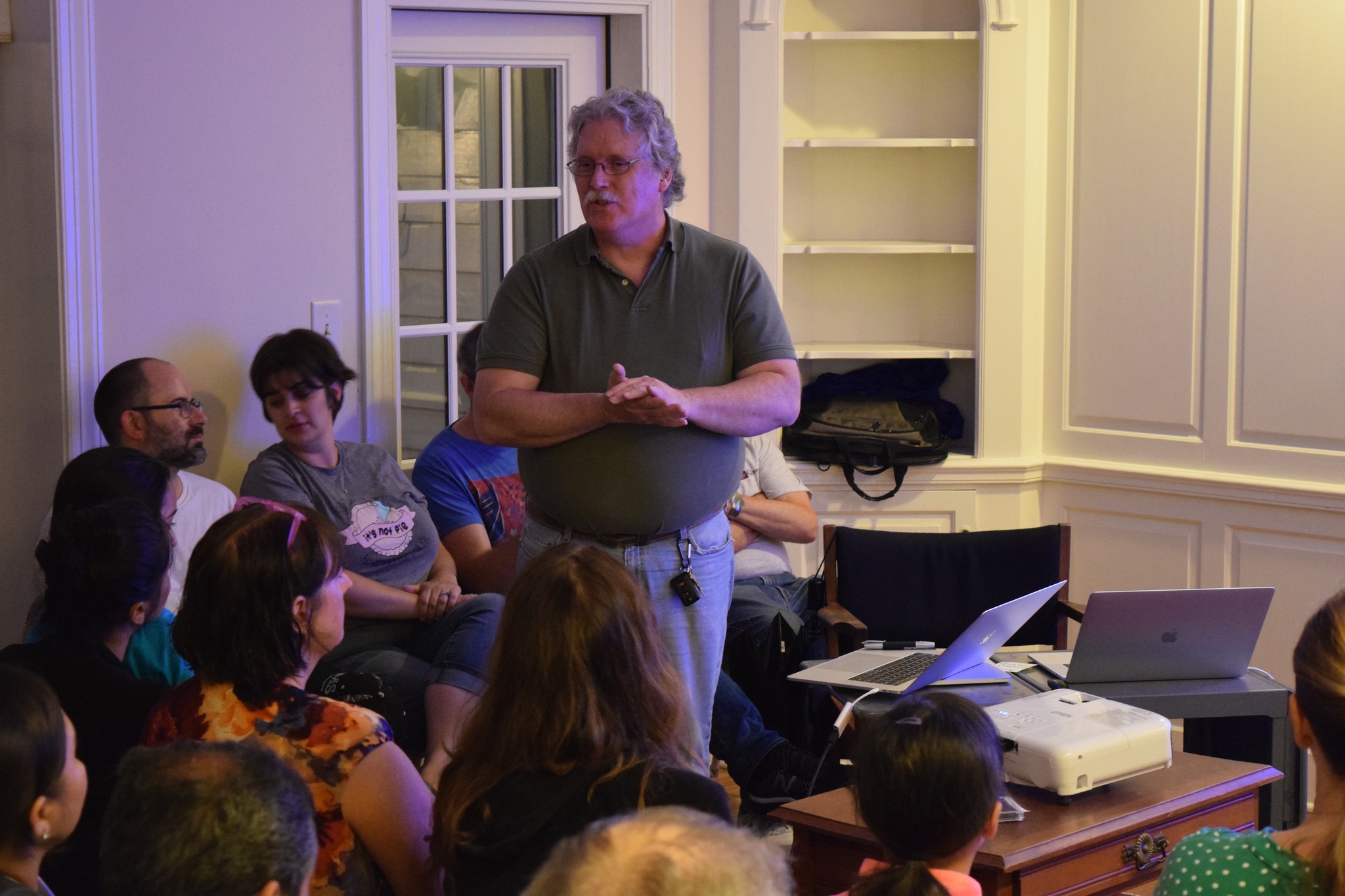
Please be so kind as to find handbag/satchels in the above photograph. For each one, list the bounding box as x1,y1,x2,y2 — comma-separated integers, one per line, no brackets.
788,397,950,502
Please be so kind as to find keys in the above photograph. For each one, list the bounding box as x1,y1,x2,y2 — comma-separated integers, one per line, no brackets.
689,572,705,596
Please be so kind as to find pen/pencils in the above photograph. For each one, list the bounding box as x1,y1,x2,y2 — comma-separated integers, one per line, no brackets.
862,640,935,650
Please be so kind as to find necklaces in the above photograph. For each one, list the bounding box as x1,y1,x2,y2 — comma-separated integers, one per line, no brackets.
522,806,800,896
324,455,349,494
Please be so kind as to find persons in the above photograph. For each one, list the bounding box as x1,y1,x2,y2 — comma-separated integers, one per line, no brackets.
97,741,320,896
453,540,733,896
144,498,445,896
468,93,801,778
412,320,827,803
0,503,173,895
1152,588,1345,896
830,689,1004,896
22,450,196,686
0,665,89,896
720,435,829,847
240,330,508,791
34,355,239,618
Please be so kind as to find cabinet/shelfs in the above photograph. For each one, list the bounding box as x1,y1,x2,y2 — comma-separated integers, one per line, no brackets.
783,29,978,360
768,749,1284,896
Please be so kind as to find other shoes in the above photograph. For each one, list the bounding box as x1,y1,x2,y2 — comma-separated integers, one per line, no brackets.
748,770,821,803
772,741,827,785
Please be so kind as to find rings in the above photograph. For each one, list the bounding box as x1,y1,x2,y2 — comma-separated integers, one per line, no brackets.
440,592,450,597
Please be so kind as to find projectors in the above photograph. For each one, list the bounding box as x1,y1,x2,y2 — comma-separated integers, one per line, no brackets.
989,689,1172,795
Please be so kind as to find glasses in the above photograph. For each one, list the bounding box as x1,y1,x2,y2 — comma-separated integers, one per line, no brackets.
233,493,308,547
132,397,204,419
567,155,641,176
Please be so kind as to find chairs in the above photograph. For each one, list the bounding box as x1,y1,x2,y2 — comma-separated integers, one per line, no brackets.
823,523,1088,728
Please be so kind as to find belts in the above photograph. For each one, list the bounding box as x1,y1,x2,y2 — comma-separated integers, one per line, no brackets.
525,501,726,549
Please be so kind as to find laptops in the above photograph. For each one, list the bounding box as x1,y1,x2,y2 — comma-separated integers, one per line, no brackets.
1028,587,1276,684
786,580,1068,695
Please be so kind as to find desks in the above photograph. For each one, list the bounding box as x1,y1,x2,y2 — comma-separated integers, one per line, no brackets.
993,654,1291,835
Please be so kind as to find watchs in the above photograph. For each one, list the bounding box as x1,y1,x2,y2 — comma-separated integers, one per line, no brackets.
727,493,744,518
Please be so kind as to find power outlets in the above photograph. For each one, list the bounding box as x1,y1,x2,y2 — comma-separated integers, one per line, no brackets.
311,300,341,357
